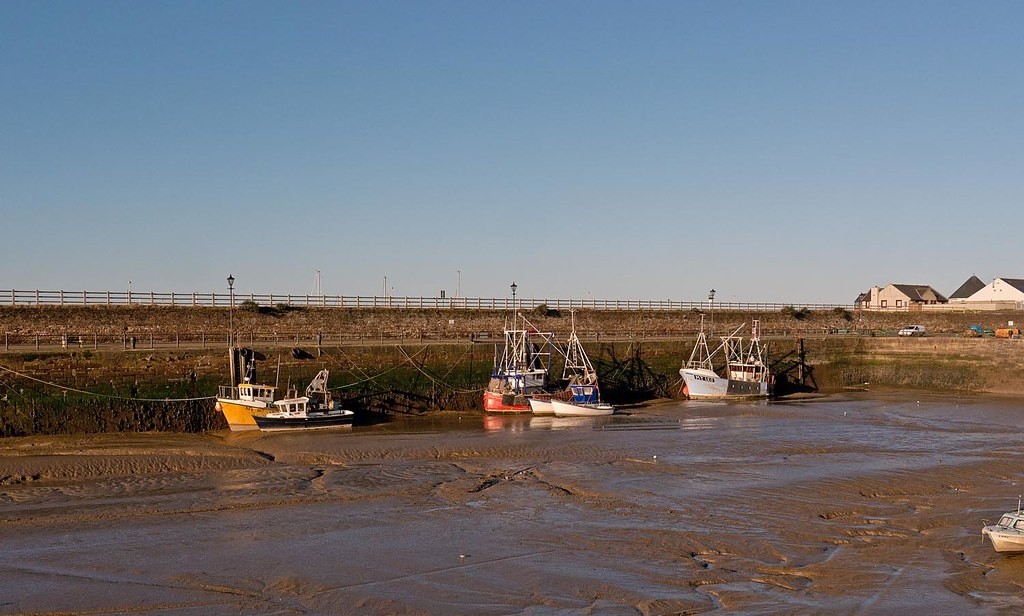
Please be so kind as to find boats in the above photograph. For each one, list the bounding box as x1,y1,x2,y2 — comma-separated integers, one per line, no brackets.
981,493,1024,554
526,394,554,415
251,397,354,434
679,338,776,400
217,370,329,431
550,384,615,417
483,312,601,413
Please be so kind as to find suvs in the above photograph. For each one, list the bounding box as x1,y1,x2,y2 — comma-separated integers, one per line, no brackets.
898,325,927,337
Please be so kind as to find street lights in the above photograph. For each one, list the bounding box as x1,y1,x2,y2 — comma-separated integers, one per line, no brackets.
708,288,717,338
510,281,518,331
227,275,235,332
